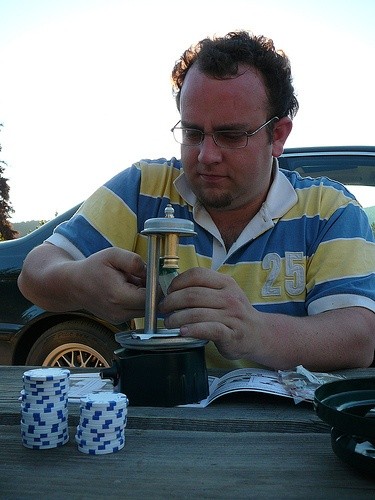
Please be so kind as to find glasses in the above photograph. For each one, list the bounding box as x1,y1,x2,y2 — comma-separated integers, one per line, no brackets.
171,116,279,149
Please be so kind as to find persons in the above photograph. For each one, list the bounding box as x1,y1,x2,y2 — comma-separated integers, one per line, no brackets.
17,30,375,373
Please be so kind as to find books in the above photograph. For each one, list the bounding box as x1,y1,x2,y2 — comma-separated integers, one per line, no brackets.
18,368,347,407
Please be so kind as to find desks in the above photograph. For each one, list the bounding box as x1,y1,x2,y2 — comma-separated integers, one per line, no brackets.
0,365,375,500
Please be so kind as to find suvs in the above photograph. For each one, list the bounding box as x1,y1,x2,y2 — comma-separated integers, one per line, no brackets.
1,146,375,369
